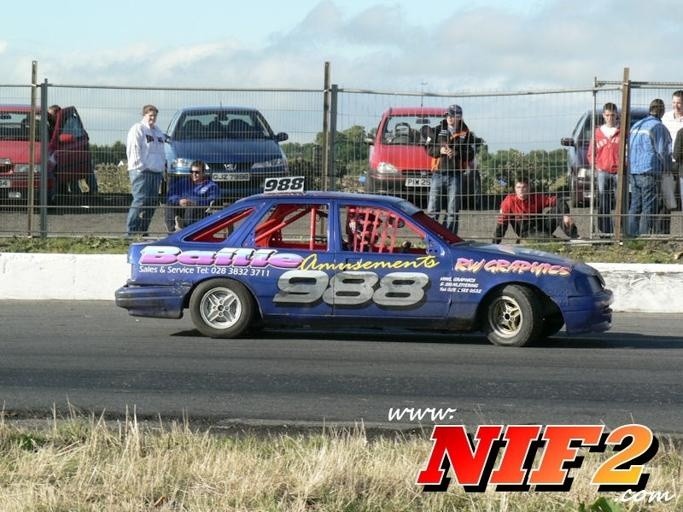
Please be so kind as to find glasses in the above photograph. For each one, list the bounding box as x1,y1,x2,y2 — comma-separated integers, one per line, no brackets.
189,171,199,174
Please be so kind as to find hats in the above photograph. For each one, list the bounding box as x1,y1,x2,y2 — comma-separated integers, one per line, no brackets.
443,104,462,117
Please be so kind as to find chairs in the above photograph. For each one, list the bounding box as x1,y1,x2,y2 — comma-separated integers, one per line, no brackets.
185,118,251,135
392,121,414,143
17,117,40,135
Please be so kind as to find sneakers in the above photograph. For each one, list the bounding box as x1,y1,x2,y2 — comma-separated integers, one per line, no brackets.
175,215,186,231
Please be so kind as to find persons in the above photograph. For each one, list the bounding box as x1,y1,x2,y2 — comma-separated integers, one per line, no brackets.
660,91,683,245
628,99,673,239
124,105,167,238
49,105,99,197
493,176,582,245
587,103,627,246
674,127,683,214
165,161,222,239
421,105,475,238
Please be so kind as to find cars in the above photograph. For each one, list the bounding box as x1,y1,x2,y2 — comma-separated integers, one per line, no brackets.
162,106,291,210
0,103,97,214
562,106,662,206
115,177,612,347
365,106,481,209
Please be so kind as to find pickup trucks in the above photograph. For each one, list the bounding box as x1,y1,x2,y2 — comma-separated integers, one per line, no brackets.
288,145,347,178
